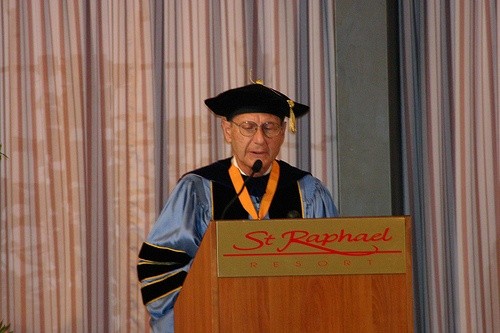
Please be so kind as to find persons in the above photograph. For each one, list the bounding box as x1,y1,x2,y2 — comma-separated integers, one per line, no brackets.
135,80,342,333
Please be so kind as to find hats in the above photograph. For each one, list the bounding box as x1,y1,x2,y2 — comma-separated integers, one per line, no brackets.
206,84,310,135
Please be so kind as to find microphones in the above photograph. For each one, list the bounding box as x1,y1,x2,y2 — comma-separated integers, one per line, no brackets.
218,159,263,220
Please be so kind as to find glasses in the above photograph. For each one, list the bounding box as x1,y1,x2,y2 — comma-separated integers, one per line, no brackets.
230,120,287,139
